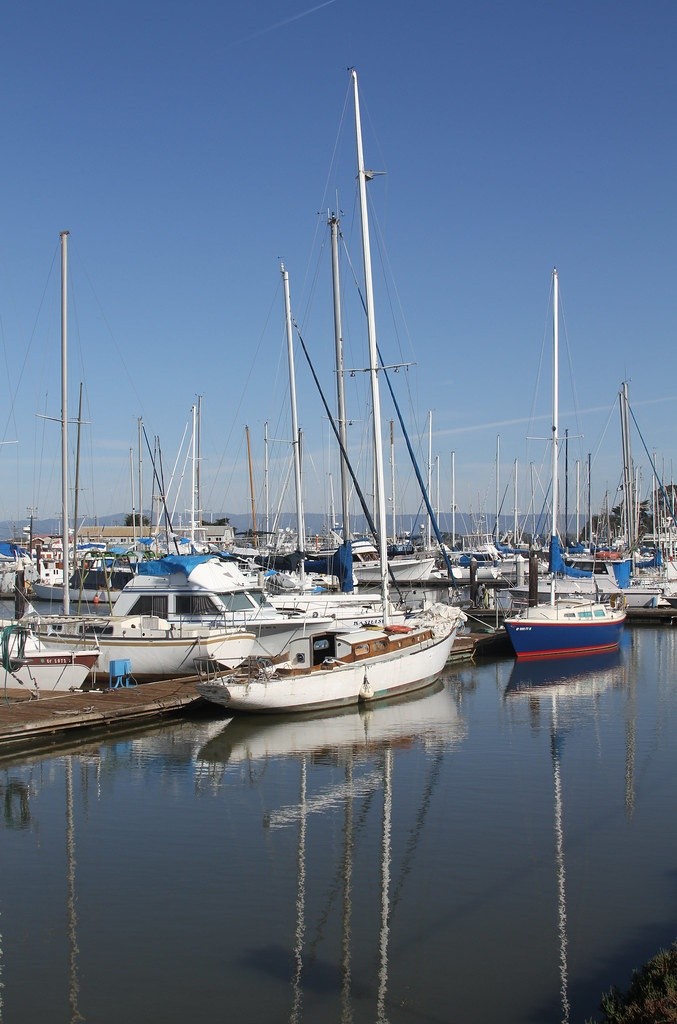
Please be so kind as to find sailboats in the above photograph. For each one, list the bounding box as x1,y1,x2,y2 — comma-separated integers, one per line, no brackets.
0,65,676,715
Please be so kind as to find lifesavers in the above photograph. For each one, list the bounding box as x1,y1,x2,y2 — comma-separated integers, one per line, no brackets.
610,593,626,609
596,552,619,559
384,625,412,635
